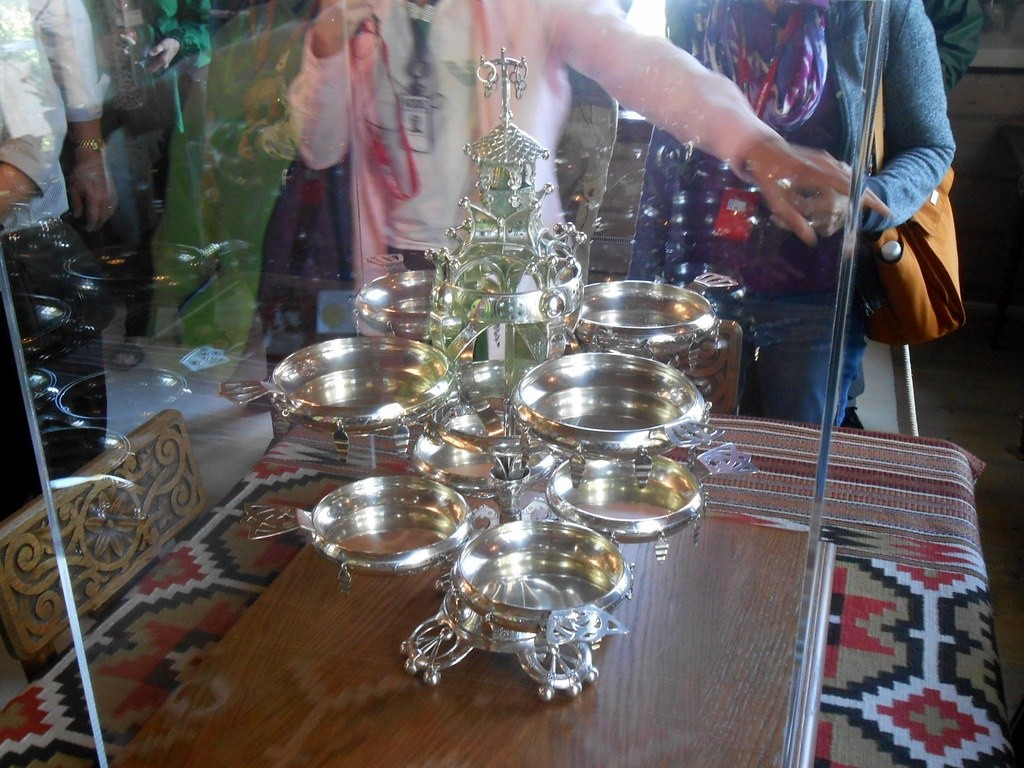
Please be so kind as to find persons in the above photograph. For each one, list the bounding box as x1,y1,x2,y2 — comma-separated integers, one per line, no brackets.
555,0,633,286
0,0,214,526
625,0,956,430
285,0,895,332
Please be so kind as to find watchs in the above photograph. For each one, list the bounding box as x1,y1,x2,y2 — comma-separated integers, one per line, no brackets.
71,137,104,152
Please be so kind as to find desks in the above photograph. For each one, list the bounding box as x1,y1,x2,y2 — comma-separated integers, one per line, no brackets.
1,404,1012,768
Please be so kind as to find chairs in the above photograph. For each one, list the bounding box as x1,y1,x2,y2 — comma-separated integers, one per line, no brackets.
1,406,209,685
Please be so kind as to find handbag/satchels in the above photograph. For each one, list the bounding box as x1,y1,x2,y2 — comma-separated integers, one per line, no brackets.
257,149,352,396
851,0,966,342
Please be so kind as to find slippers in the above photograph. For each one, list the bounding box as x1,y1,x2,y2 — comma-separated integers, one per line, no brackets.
109,339,148,371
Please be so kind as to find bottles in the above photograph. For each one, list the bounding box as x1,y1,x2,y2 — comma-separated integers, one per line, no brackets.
881,241,903,264
